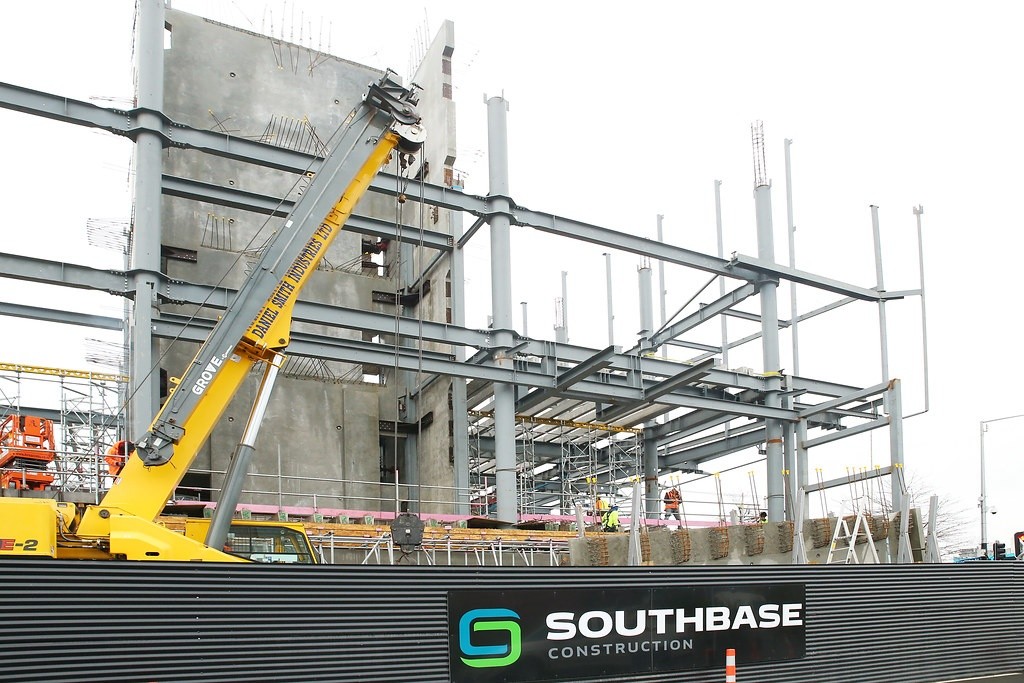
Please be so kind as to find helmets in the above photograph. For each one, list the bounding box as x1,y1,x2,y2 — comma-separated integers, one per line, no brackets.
612,503,618,507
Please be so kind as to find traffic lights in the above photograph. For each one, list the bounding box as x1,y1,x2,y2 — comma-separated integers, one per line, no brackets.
1015,532,1024,557
993,543,1006,560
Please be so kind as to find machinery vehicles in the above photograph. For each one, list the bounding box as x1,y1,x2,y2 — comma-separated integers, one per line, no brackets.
2,67,426,565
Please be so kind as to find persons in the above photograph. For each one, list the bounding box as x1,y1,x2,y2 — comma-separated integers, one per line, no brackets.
596,498,608,509
104,440,135,484
662,489,683,529
757,512,768,523
601,503,620,532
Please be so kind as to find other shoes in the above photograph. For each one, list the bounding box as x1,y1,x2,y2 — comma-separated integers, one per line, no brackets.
678,526,683,529
